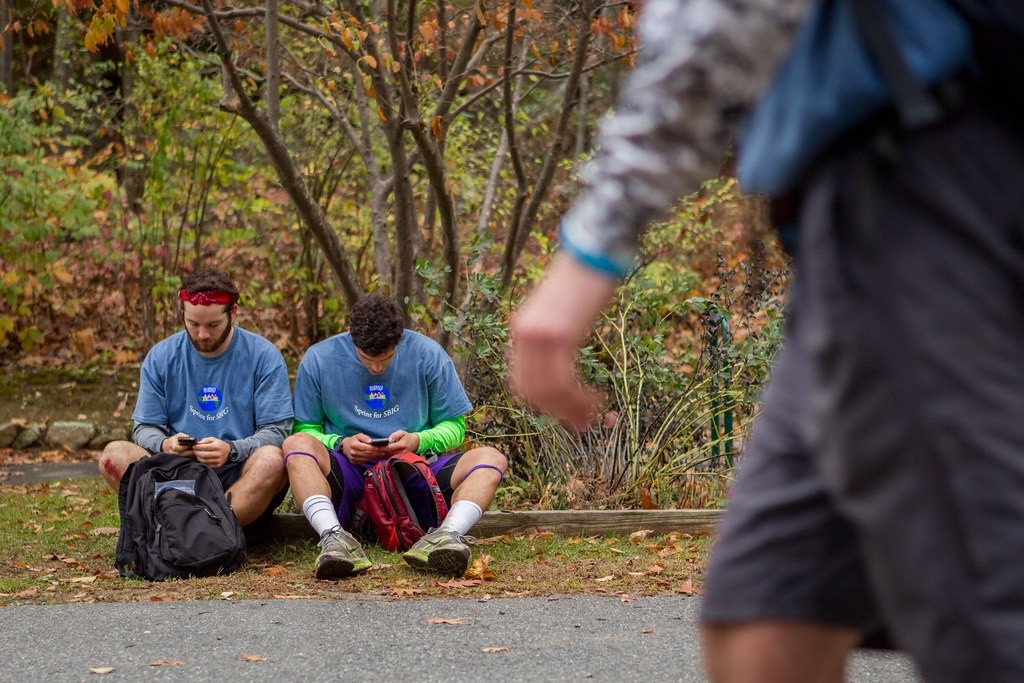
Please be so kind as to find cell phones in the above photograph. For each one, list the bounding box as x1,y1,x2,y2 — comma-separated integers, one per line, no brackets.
178,437,197,451
371,439,389,447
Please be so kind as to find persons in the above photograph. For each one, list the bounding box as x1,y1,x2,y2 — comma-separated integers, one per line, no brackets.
98,266,294,536
510,0,1024,683
281,294,508,581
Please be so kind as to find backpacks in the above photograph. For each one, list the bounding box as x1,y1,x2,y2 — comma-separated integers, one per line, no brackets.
349,452,448,554
115,452,247,583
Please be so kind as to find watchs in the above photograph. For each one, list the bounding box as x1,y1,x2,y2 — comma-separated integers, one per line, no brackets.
334,436,346,454
224,440,239,463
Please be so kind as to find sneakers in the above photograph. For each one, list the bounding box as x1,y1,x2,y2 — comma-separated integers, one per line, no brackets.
402,528,477,579
315,524,372,579
